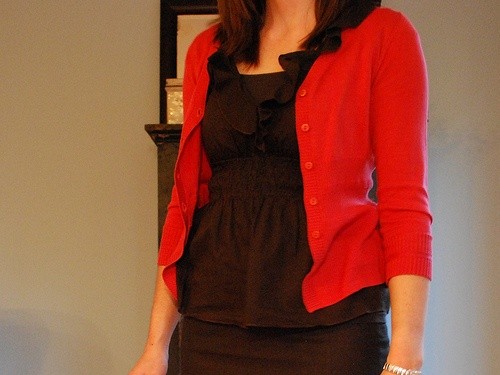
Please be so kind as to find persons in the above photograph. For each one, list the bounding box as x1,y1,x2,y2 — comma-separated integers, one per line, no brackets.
130,0,430,375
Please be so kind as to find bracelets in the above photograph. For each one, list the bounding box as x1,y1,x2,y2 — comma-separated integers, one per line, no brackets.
382,363,423,375
